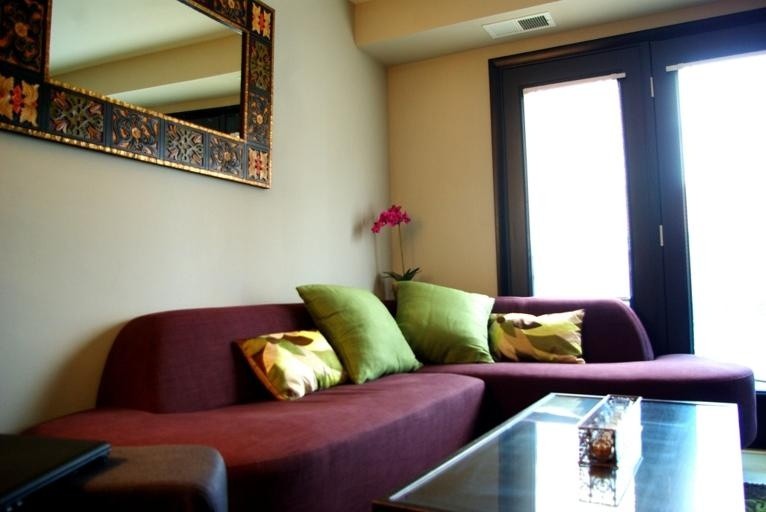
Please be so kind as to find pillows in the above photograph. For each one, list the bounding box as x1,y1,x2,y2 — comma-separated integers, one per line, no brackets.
237,329,349,400
293,282,424,385
391,279,496,368
484,307,585,367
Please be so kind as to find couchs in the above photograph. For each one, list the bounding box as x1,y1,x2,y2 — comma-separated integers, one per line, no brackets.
15,297,757,512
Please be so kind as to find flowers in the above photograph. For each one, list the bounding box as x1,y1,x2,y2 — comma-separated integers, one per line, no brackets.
370,204,422,278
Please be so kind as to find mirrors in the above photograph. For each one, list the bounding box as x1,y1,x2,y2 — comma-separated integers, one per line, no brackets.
0,1,275,188
